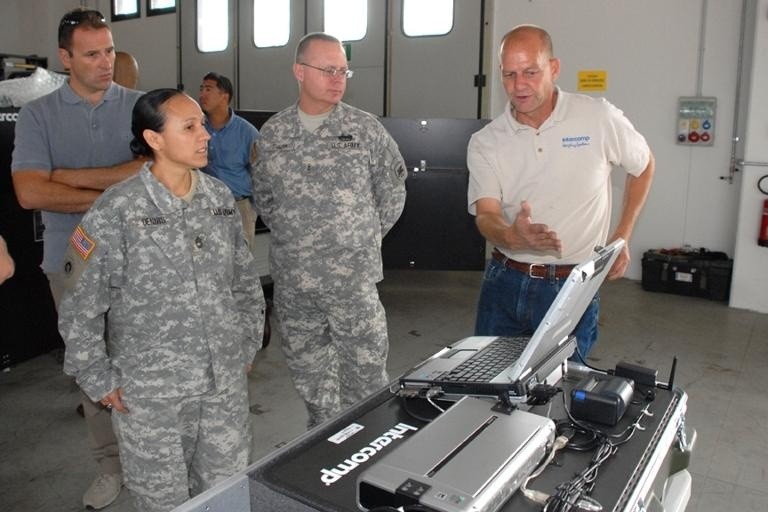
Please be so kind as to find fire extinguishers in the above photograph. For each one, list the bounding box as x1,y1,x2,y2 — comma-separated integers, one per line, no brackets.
758,174,768,247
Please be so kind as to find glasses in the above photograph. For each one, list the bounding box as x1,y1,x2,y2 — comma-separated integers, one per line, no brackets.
57,10,106,45
300,62,353,79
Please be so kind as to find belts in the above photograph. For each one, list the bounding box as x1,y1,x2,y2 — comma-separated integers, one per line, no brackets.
492,247,568,281
236,195,248,201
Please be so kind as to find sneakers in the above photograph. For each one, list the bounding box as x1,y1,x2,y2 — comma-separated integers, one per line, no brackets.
82,473,124,511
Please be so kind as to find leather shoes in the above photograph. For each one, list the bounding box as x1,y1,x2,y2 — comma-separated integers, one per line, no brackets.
263,299,273,347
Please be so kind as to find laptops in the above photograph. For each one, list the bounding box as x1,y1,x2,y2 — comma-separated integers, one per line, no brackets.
400,238,625,406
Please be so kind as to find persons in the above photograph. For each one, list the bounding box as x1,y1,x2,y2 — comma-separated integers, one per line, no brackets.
0,232,18,291
250,33,409,432
113,51,138,91
11,8,149,511
466,25,656,362
55,87,265,511
199,73,260,259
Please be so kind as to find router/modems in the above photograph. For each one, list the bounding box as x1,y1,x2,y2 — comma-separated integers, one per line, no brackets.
615,357,678,392
